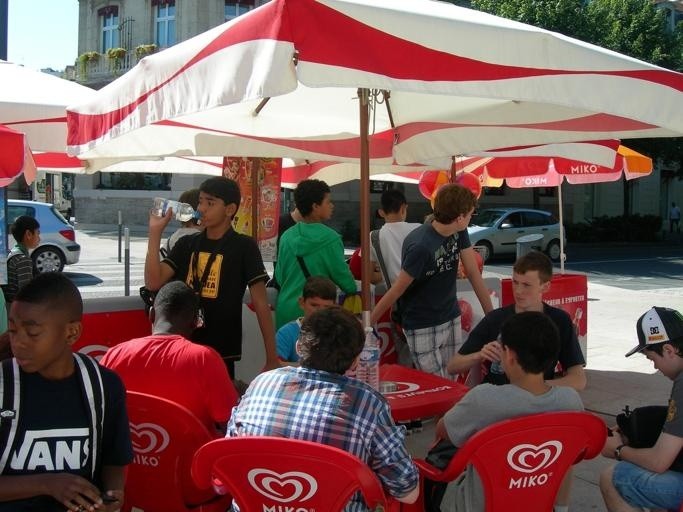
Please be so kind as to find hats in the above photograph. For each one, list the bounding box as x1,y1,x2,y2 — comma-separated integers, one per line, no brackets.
625,306,683,357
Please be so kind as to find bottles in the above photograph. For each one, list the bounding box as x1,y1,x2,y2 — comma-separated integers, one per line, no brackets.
356,327,381,392
151,196,201,223
489,333,505,376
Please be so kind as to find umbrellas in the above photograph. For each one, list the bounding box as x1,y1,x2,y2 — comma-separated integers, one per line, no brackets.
65,0,683,310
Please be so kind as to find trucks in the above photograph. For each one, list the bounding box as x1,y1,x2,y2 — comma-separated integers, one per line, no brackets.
32,170,72,223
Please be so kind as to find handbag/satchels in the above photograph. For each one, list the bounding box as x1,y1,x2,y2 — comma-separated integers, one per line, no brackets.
616,406,683,472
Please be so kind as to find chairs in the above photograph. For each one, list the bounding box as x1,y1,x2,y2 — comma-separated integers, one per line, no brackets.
120,391,234,512
402,409,608,512
458,353,564,387
191,437,400,512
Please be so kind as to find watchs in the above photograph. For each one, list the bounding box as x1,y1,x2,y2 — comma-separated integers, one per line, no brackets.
615,444,626,461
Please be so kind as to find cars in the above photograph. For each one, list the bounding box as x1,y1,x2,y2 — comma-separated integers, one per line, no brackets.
467,207,567,261
7,199,82,276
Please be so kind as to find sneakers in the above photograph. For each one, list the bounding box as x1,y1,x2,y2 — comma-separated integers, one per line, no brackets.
396,422,412,434
412,420,423,432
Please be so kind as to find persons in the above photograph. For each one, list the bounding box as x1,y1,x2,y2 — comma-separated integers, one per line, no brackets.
668,202,681,233
2,215,42,303
223,306,421,512
0,271,134,512
599,306,682,512
422,311,586,511
142,175,358,385
358,185,495,425
98,280,242,438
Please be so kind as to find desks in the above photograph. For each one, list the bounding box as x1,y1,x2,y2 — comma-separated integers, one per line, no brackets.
344,363,471,424
